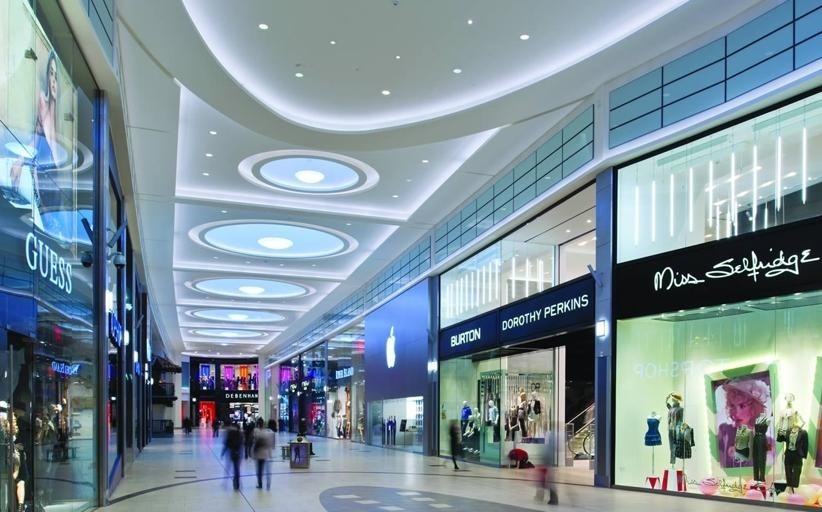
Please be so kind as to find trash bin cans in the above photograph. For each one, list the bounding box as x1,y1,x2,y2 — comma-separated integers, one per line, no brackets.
289,437,310,469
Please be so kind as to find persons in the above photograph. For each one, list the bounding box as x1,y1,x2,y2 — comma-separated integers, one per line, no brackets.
212,411,286,459
184,416,191,434
335,413,348,439
460,400,481,454
507,448,535,468
11,457,27,512
220,423,243,489
249,417,275,490
10,50,59,238
547,488,558,505
645,392,695,464
734,393,809,487
488,400,500,441
297,428,316,455
718,379,775,467
199,373,256,391
503,382,541,443
34,398,69,462
449,425,461,466
